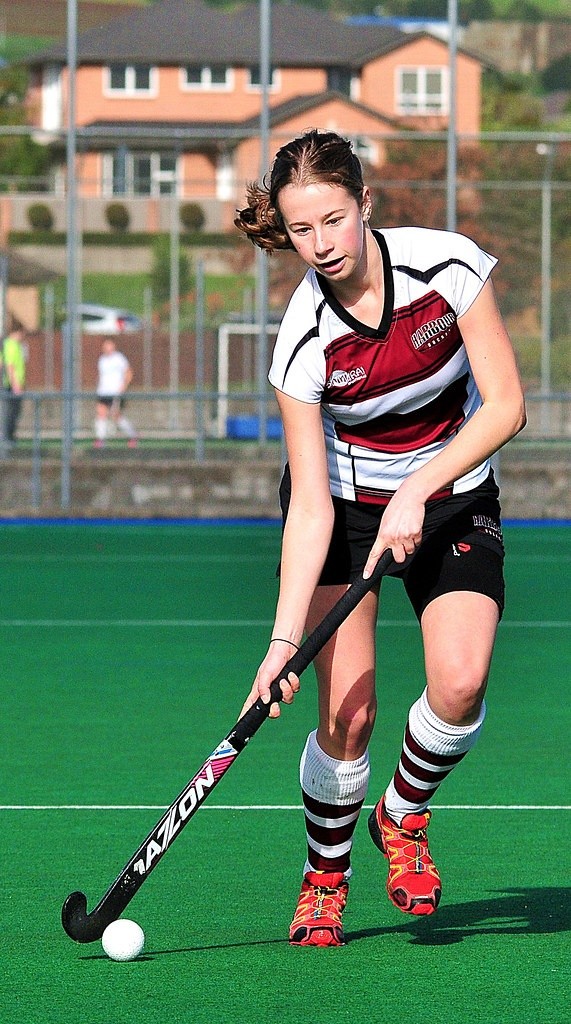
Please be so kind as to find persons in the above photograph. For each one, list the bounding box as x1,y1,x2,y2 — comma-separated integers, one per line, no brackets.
93,337,138,448
234,125,529,949
1,318,26,453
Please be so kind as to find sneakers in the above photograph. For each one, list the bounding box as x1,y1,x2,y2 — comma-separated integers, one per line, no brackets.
288,872,349,946
368,794,442,915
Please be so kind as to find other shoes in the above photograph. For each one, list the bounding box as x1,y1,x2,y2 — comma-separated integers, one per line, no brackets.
92,441,103,448
126,436,136,448
0,437,17,454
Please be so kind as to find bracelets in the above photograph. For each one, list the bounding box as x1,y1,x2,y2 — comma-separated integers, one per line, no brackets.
268,637,301,653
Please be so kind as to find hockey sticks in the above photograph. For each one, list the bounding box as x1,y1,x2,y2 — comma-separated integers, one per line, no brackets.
57,548,396,943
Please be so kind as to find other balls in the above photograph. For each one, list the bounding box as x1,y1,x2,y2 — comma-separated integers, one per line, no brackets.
99,916,146,961
